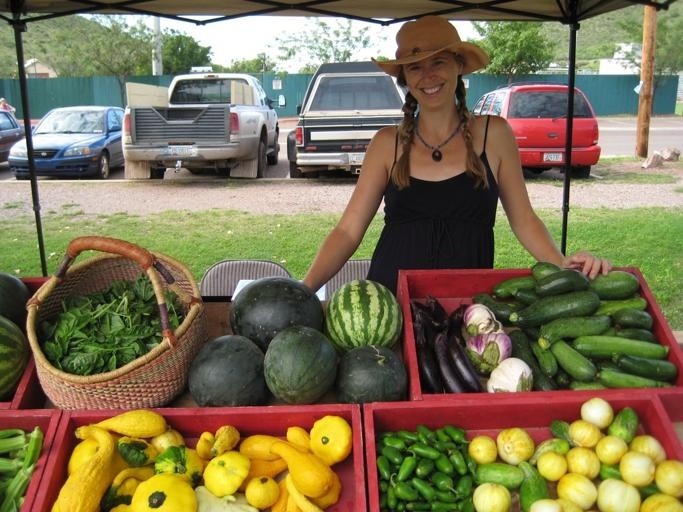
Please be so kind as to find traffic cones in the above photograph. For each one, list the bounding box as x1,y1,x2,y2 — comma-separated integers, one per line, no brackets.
468,398,683,511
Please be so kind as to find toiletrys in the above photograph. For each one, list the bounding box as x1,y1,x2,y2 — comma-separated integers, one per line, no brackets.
376,424,476,512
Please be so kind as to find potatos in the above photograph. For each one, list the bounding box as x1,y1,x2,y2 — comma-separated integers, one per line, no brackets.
470,261,679,390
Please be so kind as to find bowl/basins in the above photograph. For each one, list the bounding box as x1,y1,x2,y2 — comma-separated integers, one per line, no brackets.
414,119,465,164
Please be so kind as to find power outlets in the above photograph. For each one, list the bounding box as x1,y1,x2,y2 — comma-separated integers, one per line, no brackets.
122,68,281,177
290,62,413,175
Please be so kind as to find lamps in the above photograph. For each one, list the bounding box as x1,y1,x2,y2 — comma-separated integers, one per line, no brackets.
324,260,372,300
200,259,292,296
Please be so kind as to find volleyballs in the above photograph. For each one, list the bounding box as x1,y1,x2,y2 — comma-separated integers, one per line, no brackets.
409,293,481,395
461,302,534,393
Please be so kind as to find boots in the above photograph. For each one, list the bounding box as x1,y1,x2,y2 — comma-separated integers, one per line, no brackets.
0,104,31,164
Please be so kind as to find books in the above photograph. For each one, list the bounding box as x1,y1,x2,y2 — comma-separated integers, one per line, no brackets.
0,278,51,410
397,268,683,400
0,409,63,512
31,404,367,512
363,395,683,512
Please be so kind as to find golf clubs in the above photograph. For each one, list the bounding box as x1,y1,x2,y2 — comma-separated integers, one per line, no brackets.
189,277,409,408
1,271,29,401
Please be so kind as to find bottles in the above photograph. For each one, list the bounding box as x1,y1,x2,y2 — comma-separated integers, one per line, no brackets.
371,15,491,75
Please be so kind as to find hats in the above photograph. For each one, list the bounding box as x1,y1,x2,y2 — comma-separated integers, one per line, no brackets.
9,106,123,180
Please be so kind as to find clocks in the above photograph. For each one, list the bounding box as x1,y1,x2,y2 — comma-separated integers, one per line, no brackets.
37,272,183,376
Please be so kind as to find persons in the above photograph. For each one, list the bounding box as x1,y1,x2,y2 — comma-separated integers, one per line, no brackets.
301,17,613,292
0,98,15,113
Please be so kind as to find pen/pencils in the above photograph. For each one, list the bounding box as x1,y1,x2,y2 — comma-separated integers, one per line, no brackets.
475,85,598,180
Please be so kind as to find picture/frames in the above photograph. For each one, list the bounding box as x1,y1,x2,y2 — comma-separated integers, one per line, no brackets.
43,301,683,449
